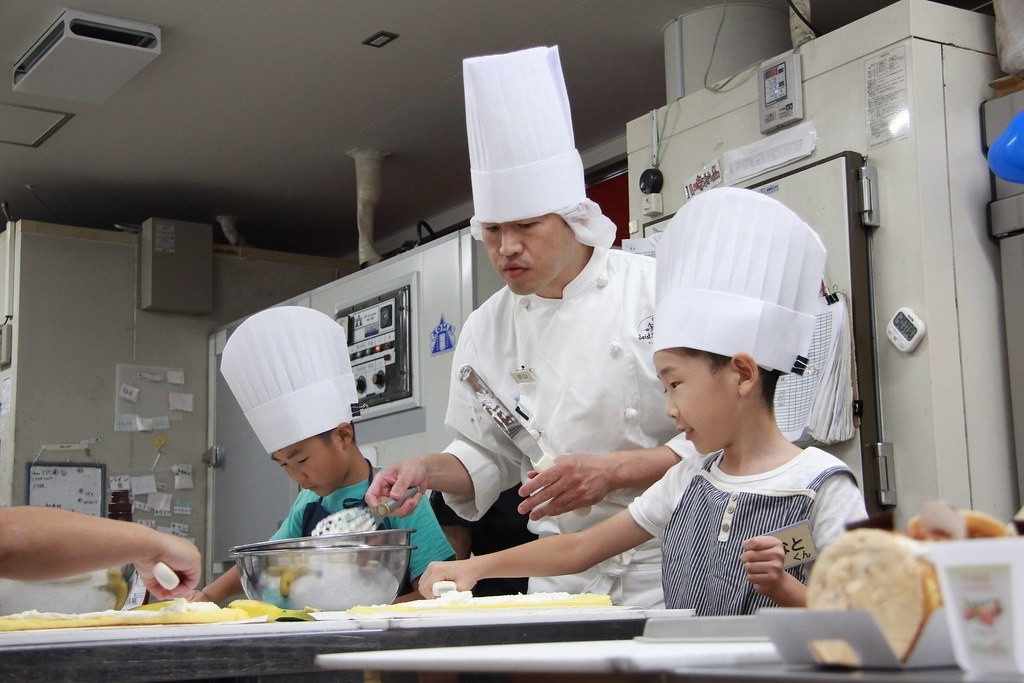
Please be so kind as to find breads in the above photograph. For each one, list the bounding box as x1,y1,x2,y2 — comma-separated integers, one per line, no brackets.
806,530,930,668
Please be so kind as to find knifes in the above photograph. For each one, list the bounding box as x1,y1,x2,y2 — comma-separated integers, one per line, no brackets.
431,580,457,600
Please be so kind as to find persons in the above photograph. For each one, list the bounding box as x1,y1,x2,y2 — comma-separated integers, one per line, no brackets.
182,381,458,608
362,151,696,610
416,274,871,617
0,506,202,602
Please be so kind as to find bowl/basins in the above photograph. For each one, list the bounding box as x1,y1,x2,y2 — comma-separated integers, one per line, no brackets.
227,526,419,612
910,535,1024,676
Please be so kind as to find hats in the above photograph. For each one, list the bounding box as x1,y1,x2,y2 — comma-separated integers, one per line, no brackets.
220,306,360,455
463,44,587,223
652,187,827,375
